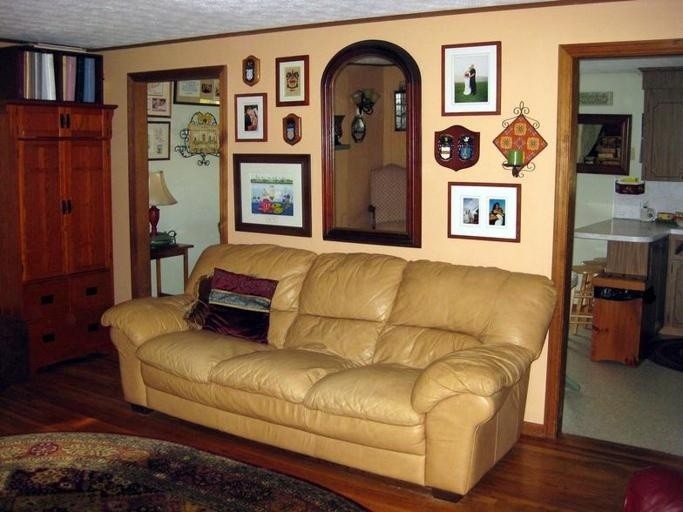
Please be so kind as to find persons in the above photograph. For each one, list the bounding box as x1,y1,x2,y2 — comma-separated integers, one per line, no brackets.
493,203,504,224
463,65,476,95
245,106,258,130
155,99,165,111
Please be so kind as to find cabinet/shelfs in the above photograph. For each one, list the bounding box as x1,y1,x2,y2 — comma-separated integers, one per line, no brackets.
637,65,682,182
0,97,117,375
659,233,682,336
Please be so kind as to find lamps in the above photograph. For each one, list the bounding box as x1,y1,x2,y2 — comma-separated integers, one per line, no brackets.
351,87,380,115
148,170,178,237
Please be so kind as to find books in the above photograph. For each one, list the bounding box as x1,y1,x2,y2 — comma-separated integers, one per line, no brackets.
1,42,103,104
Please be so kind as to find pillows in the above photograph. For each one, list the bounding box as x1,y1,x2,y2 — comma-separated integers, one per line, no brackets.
200,266,278,345
184,273,213,330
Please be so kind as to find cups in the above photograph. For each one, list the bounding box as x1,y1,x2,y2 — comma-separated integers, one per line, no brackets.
640,208,653,223
508,149,523,165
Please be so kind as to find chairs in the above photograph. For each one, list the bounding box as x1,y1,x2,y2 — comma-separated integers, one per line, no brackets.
368,162,405,233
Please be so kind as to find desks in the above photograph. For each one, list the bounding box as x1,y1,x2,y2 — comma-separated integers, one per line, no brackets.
149,243,192,296
573,216,670,369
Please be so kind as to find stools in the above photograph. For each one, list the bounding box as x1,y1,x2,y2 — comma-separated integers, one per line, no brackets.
568,255,607,336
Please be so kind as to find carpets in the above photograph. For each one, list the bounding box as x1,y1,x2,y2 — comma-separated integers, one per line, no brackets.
645,335,683,372
0,431,371,512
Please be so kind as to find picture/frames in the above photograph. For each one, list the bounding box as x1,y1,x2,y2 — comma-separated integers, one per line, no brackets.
172,78,219,106
577,113,631,176
232,152,313,240
446,181,520,243
233,94,268,142
146,82,171,118
440,41,500,117
146,120,170,160
274,55,311,110
349,116,366,144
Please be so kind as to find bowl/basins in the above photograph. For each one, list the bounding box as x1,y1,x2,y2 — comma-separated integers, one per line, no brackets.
673,215,683,227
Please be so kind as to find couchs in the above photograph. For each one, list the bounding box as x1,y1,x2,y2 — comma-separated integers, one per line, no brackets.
100,242,556,503
